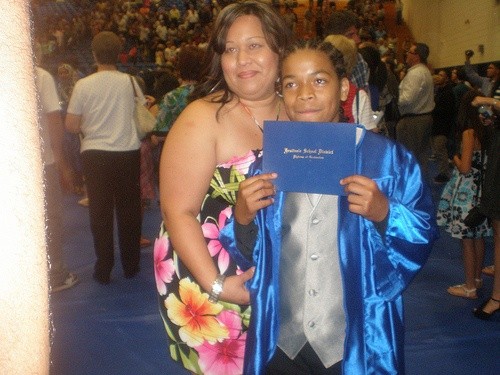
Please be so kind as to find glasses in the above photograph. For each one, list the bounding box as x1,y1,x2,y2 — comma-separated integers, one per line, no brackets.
408,50,419,55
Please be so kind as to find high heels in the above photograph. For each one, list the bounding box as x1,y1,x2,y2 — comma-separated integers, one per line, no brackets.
473,296,500,320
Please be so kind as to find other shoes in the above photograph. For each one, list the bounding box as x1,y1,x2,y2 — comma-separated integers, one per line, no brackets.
481,265,496,275
447,278,481,299
432,175,449,183
140,237,152,248
93,268,110,284
78,199,90,207
124,266,141,279
52,271,79,293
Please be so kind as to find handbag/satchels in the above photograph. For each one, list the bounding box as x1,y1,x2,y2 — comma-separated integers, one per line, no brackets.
463,206,487,228
130,75,159,141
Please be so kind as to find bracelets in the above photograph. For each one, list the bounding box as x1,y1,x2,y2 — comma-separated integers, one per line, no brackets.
208,274,225,304
492,99,499,107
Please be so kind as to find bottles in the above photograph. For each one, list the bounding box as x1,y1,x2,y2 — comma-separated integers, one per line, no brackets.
477,104,496,126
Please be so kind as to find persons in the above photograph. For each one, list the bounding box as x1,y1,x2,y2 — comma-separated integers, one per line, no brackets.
30,0,230,248
430,69,456,184
33,65,79,294
470,75,500,320
437,91,494,299
154,1,292,375
218,38,437,375
64,32,148,285
434,50,500,165
229,0,412,139
396,42,436,188
